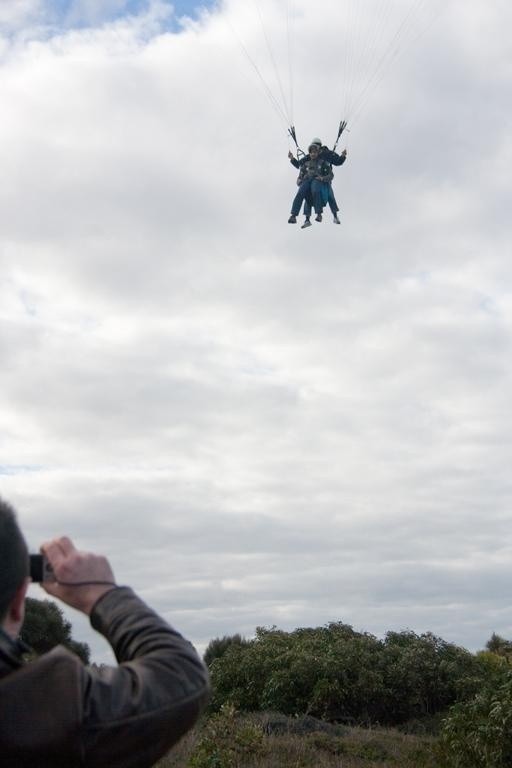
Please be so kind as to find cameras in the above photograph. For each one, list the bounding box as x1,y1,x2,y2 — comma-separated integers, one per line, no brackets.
28,553,55,583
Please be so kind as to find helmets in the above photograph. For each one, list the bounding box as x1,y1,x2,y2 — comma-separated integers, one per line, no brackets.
308,138,321,150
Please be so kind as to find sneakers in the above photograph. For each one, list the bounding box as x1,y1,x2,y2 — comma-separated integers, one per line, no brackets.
288,216,340,229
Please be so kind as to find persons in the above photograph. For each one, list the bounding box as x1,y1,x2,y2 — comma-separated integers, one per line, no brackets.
1,499,210,767
288,138,347,229
